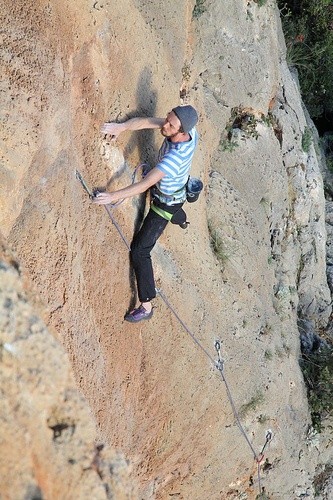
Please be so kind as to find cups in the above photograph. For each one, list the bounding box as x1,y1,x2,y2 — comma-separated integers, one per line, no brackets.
187,180,204,203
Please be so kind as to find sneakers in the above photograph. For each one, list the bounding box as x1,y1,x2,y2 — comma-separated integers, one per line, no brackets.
124,303,153,323
178,221,190,229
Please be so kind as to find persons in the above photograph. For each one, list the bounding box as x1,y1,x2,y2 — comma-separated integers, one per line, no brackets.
93,105,199,322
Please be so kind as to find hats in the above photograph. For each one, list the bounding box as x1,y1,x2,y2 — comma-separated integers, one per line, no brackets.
172,103,198,136
